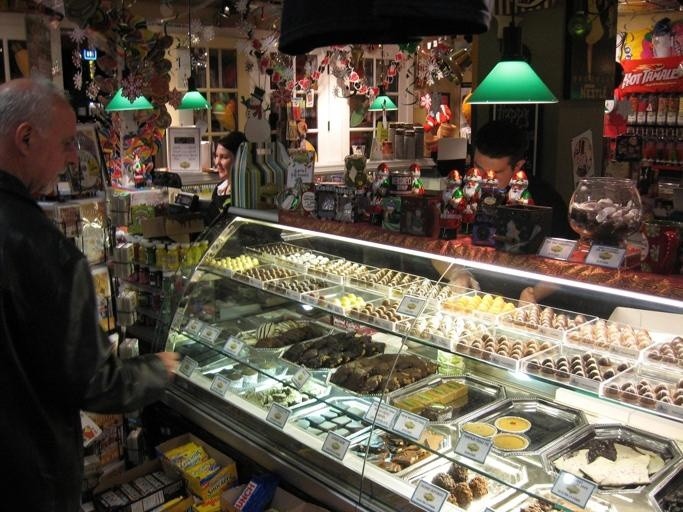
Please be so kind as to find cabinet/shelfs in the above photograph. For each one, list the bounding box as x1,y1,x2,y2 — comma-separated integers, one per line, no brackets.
113,257,232,354
605,86,683,229
149,202,683,512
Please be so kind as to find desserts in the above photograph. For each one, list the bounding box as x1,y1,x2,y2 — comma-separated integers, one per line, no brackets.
172,241,683,512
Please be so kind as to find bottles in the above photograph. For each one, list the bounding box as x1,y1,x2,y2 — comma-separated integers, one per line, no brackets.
470,186,509,247
620,91,683,162
387,121,425,161
113,230,209,330
565,174,644,270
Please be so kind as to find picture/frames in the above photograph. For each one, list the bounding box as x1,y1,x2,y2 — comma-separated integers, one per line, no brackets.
166,127,201,174
57,123,111,202
492,105,539,176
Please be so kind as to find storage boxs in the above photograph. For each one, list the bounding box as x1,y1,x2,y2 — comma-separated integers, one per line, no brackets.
80,431,334,512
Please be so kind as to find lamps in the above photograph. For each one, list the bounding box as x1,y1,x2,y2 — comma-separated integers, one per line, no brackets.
102,0,154,112
435,47,471,86
465,0,561,104
177,2,211,110
367,45,399,112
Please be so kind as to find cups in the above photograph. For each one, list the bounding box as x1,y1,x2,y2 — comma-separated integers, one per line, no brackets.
641,221,682,276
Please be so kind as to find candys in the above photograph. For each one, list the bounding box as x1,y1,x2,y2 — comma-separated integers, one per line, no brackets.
98,26,173,185
568,197,643,241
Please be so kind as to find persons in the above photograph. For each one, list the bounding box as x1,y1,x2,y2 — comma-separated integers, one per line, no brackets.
430,123,580,307
0,76,180,512
208,130,247,218
